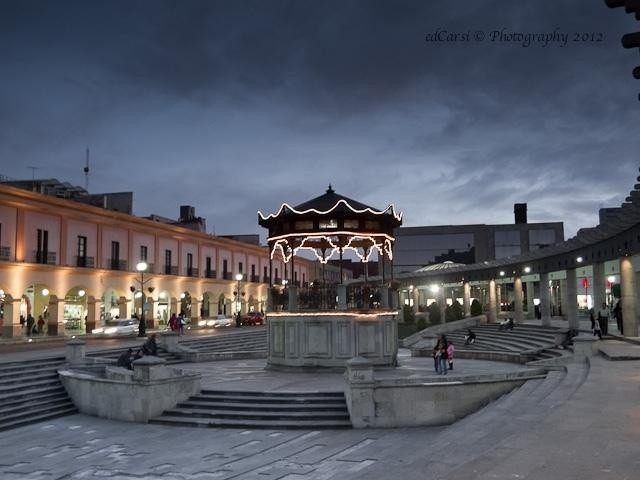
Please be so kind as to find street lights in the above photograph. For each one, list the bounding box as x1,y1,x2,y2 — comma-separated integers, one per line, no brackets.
556,285,559,315
233,273,246,328
607,276,616,309
281,280,289,289
550,285,554,315
131,259,153,339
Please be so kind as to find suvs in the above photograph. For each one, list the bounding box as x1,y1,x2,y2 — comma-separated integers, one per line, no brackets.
239,312,265,326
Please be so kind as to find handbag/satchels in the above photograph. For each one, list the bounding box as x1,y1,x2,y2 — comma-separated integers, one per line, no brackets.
180,318,186,325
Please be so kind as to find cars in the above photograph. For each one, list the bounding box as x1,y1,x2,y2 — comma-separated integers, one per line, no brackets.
91,318,141,337
160,317,192,333
197,315,236,329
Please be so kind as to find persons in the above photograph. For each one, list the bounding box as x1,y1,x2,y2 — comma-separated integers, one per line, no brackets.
463,330,472,344
506,318,514,330
592,318,603,340
613,300,622,330
230,312,238,329
466,332,476,345
116,348,132,369
141,333,158,357
130,350,143,367
431,334,455,376
20,315,25,333
25,313,35,337
37,315,45,334
498,317,508,332
174,313,185,337
165,313,177,332
597,302,611,336
